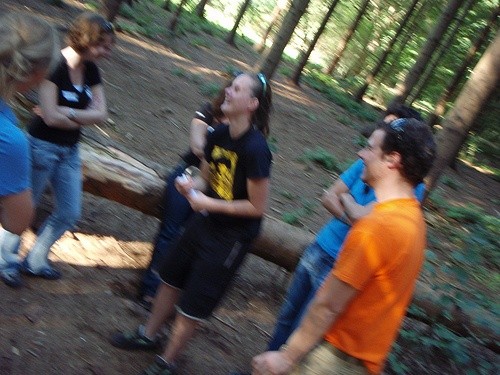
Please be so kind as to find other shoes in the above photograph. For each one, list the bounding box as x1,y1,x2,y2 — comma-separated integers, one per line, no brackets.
0,252,22,287
22,258,61,279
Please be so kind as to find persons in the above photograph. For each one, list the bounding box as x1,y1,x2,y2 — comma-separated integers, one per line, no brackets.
0,11,55,237
0,10,118,287
264,102,427,355
136,71,244,314
109,71,274,375
249,118,438,375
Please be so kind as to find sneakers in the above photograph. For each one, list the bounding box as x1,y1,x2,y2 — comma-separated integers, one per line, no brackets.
143,355,175,375
110,328,162,351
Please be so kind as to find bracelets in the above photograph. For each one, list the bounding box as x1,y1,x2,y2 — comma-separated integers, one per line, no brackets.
339,213,348,221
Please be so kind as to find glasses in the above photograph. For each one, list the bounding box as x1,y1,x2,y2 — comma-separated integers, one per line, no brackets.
258,73,268,96
104,21,115,33
390,117,408,139
